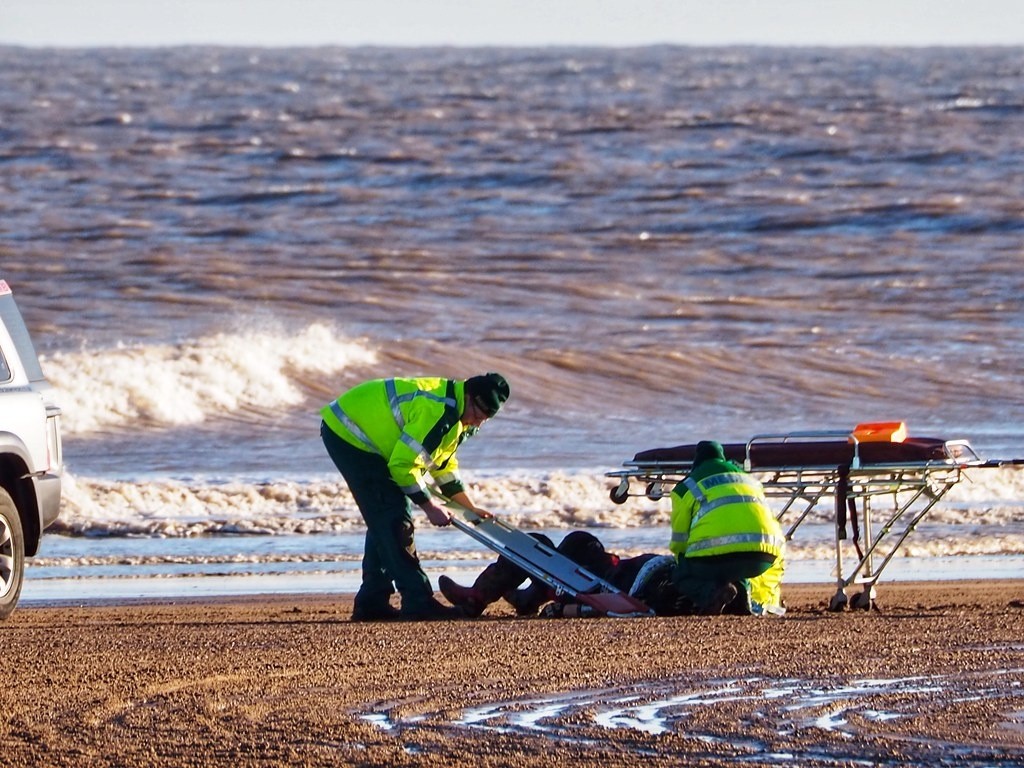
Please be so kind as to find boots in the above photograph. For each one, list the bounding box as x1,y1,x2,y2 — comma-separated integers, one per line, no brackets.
734,589,752,615
439,563,514,617
502,581,551,614
706,583,737,615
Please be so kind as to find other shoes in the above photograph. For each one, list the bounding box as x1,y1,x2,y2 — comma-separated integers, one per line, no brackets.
401,596,463,619
351,603,401,619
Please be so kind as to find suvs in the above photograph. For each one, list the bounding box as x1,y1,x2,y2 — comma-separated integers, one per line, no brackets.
0,280,65,624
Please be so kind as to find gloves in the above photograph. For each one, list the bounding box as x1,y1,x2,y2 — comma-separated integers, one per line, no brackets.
539,603,564,618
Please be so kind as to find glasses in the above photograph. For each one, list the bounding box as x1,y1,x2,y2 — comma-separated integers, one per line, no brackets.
469,396,483,423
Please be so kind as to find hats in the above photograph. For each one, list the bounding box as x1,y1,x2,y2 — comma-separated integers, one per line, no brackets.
464,373,509,417
691,440,726,466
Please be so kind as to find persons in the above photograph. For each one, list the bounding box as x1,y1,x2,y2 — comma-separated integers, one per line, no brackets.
438,441,779,617
321,372,510,619
